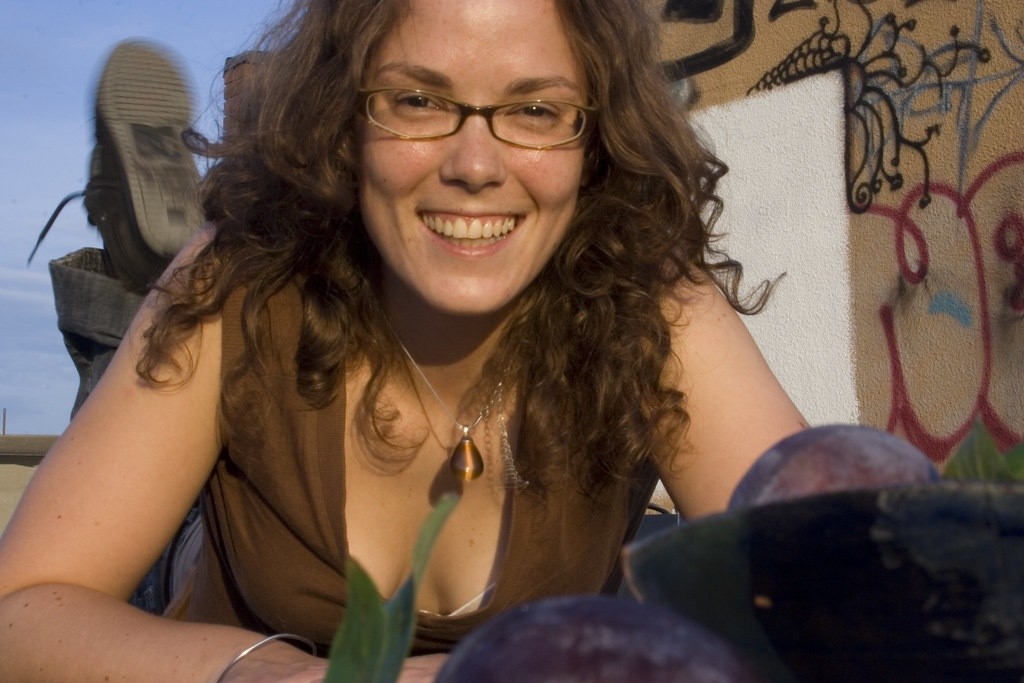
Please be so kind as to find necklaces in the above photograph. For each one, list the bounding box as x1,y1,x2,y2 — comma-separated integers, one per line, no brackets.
384,303,511,482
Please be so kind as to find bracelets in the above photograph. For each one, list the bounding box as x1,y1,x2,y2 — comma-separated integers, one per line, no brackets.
216,633,317,683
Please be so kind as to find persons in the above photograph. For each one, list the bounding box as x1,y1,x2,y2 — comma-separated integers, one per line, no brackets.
2,0,812,682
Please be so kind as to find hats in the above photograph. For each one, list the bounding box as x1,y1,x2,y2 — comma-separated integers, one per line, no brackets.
618,482,1024,683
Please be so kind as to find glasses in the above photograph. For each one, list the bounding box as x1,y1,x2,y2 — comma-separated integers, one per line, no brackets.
358,87,598,150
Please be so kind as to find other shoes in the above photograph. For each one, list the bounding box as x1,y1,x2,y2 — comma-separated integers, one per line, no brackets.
26,39,207,291
